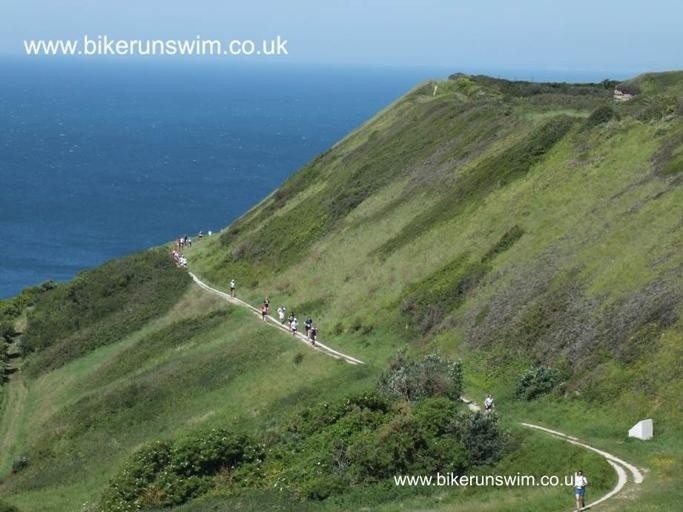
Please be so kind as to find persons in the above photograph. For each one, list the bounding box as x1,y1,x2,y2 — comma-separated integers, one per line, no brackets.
172,230,211,267
230,279,237,297
259,296,318,345
484,394,494,413
574,470,588,509
